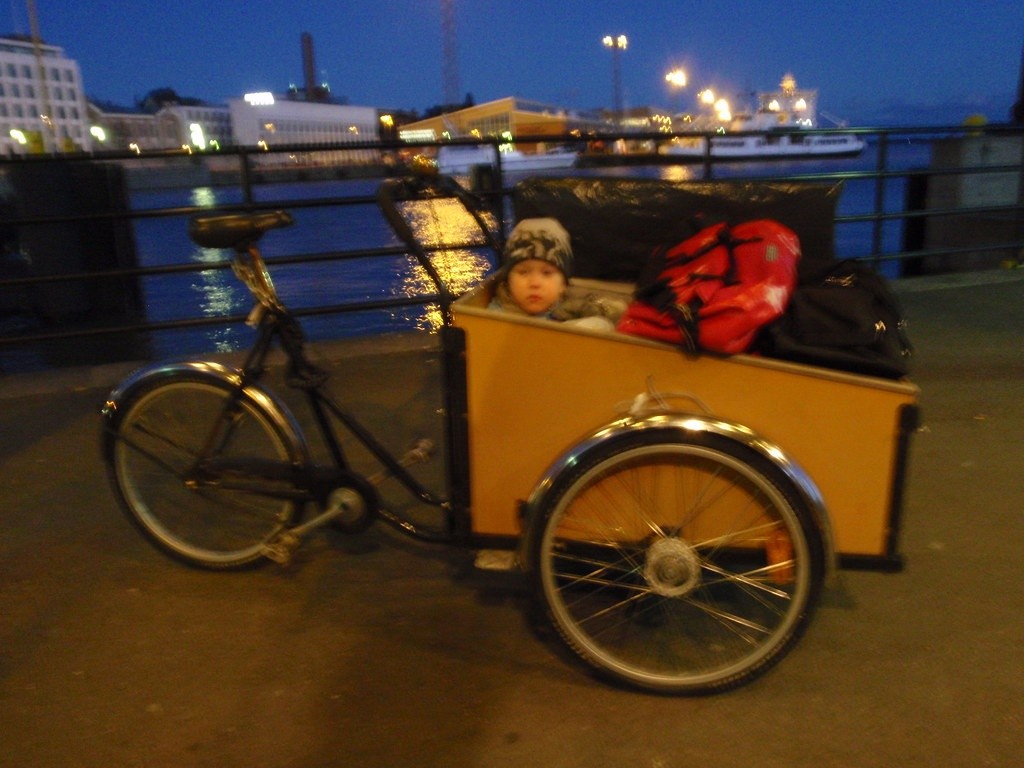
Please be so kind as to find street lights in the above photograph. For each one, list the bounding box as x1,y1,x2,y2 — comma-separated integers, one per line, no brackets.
604,33,631,128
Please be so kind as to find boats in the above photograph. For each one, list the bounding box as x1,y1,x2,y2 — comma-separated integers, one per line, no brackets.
432,121,867,176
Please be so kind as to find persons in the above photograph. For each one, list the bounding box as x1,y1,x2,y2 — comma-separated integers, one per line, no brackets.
488,217,629,331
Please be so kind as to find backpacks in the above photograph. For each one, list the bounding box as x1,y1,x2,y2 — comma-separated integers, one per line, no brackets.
617,218,799,357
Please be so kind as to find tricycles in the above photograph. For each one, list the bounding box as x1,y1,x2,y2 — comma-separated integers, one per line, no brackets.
97,165,929,701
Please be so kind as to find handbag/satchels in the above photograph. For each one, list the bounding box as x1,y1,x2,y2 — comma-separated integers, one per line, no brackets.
747,255,915,381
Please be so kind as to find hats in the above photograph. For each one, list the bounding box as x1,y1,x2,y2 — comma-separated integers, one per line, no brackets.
504,217,573,272
